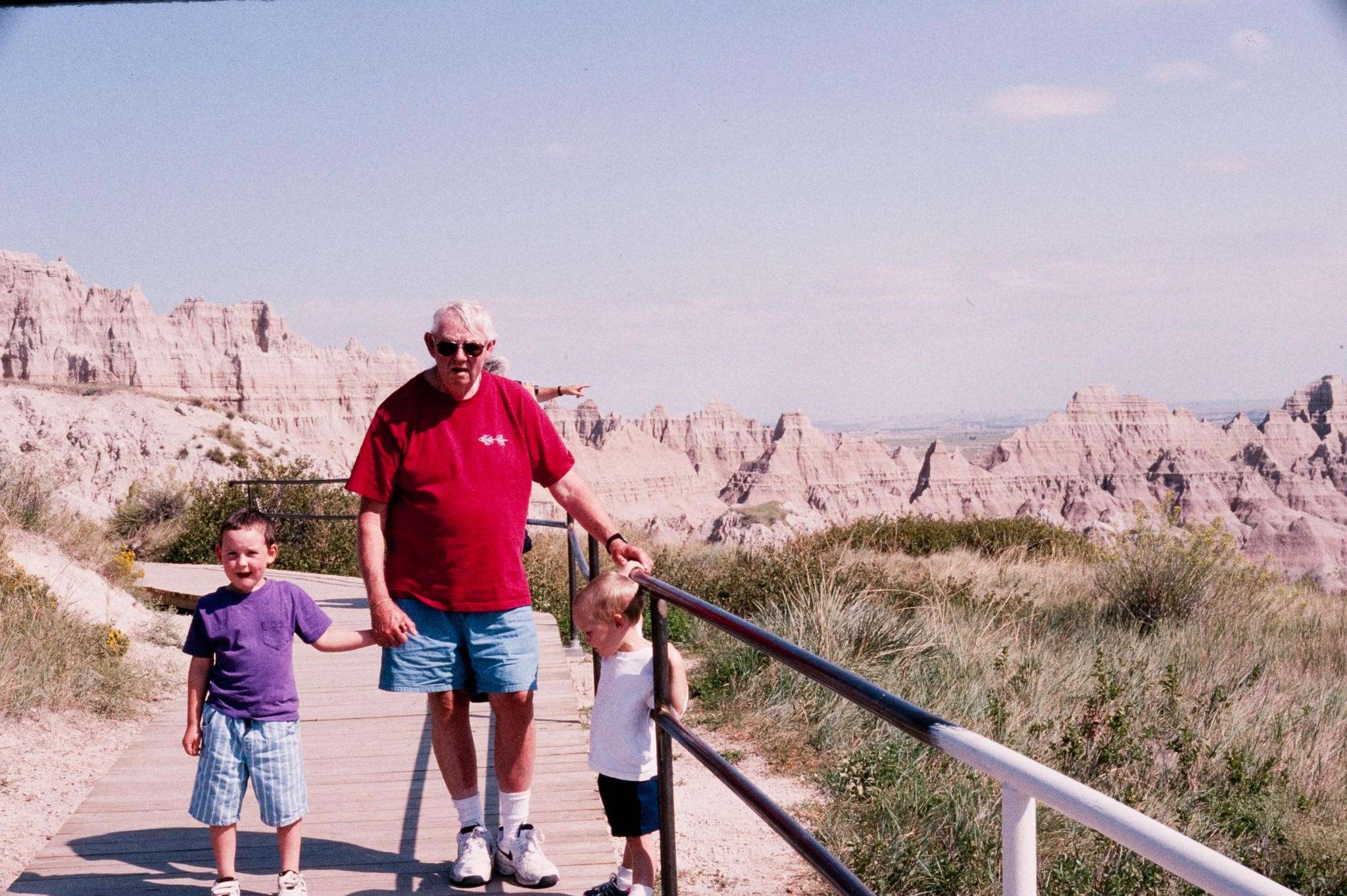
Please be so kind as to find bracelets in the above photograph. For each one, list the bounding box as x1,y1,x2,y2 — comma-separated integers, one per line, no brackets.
605,531,629,554
557,386,563,396
369,598,393,616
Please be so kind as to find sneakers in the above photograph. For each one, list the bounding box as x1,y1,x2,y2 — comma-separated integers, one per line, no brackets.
449,826,494,884
211,878,240,896
583,872,631,896
496,820,560,885
277,871,307,896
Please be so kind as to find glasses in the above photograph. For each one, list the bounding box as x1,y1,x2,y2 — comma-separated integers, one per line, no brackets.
433,333,487,357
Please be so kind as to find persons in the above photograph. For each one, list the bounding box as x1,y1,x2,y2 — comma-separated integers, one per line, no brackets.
479,356,591,408
572,570,689,896
341,300,659,891
181,509,409,896
